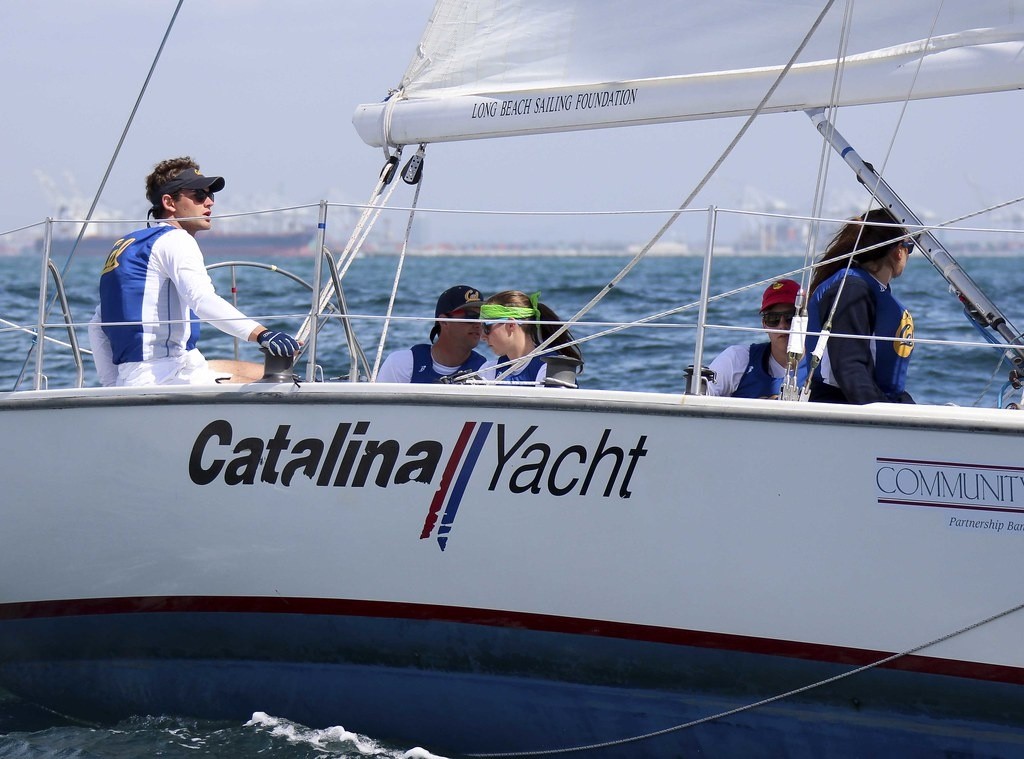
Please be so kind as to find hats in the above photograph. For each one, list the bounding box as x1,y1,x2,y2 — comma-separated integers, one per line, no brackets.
150,169,225,212
433,285,487,318
759,279,801,314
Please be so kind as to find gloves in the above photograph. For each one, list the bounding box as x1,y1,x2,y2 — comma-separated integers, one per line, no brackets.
682,366,717,383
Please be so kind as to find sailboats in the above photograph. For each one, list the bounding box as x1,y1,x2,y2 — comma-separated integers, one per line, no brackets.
0,0,1024,759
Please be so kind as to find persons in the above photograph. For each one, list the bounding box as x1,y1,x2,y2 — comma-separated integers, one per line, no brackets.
700,279,829,404
376,285,486,384
478,290,583,388
805,207,915,405
88,156,304,388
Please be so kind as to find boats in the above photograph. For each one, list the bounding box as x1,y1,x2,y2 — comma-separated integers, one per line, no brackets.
21,202,315,258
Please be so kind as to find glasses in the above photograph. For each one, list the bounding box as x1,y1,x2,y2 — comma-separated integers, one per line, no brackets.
903,242,915,254
444,310,481,324
179,187,214,203
762,310,794,327
481,317,522,334
